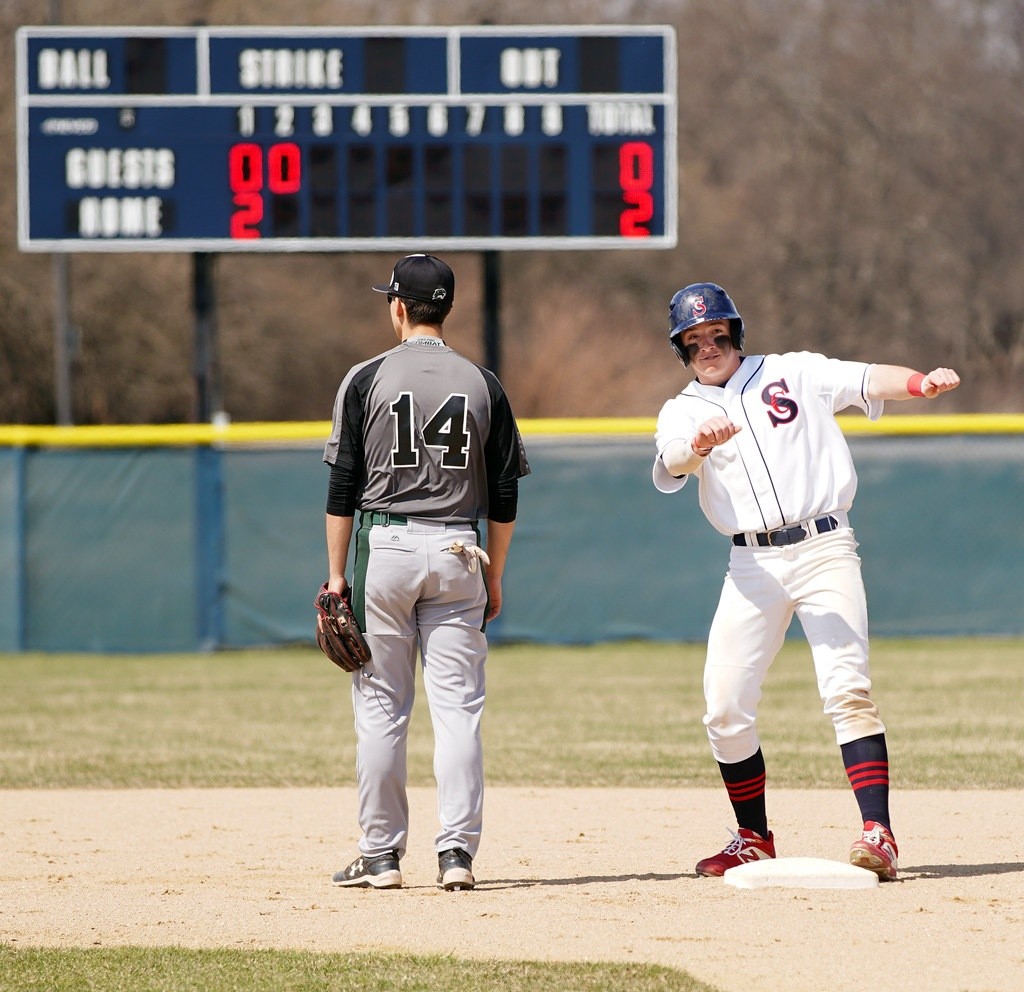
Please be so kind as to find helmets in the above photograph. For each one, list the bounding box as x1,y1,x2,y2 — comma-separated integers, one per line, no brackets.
669,282,744,370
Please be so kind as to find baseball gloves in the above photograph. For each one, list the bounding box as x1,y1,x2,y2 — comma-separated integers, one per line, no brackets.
314,582,372,673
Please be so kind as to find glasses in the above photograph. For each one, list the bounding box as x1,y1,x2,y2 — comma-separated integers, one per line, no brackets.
387,293,398,304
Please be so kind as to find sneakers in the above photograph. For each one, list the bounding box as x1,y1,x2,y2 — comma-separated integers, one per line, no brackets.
695,828,776,877
436,846,475,890
332,848,402,889
849,820,899,881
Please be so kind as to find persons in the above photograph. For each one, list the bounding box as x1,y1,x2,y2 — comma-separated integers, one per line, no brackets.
316,254,532,894
647,282,962,883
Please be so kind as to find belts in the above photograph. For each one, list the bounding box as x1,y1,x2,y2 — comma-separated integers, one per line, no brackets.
372,512,471,527
734,516,838,546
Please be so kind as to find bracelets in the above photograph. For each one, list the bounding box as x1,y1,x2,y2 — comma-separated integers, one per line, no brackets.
907,373,927,397
693,433,712,452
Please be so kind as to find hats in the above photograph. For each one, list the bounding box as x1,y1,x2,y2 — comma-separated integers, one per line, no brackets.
372,254,455,303
